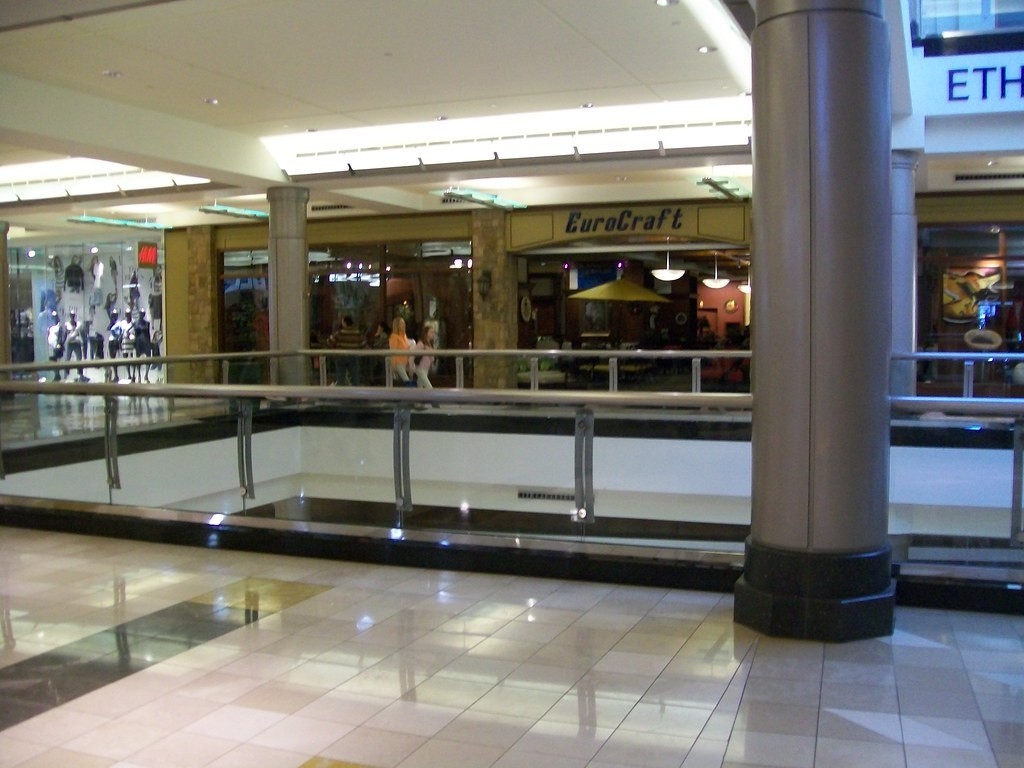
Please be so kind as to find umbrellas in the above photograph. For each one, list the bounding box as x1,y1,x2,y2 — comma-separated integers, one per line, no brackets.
568,279,670,303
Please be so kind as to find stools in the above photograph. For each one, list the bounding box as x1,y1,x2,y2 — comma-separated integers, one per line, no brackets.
963,329,1002,380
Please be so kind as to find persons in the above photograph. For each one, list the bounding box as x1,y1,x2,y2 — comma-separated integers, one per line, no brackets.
327,309,448,411
45,310,150,383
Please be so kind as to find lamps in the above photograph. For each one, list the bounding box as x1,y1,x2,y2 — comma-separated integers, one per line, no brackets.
686,164,753,202
200,198,269,219
445,184,527,212
66,210,174,230
702,252,730,288
651,250,685,281
